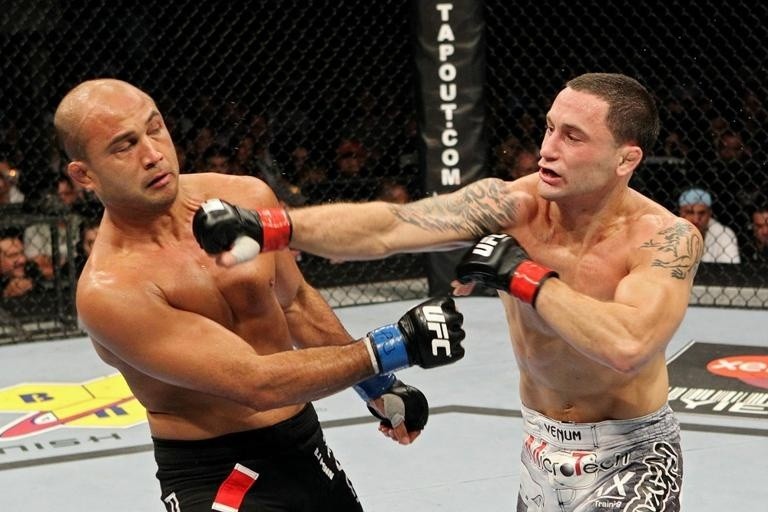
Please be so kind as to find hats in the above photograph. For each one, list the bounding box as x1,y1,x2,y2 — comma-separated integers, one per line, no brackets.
677,188,712,208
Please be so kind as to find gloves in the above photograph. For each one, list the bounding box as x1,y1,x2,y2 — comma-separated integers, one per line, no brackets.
351,372,429,433
454,232,561,309
366,292,466,375
192,197,293,266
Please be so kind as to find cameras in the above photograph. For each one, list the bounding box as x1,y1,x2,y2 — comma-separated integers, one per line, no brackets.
23,258,38,279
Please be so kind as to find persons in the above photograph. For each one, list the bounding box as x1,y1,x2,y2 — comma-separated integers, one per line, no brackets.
2,109,104,333
493,95,766,265
50,77,468,509
168,91,425,280
191,69,707,509
